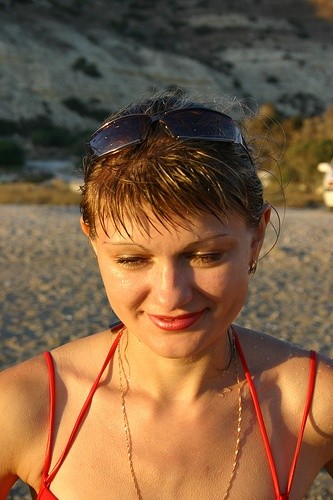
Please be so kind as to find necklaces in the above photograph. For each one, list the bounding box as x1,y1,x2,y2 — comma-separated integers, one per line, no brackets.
117,328,242,500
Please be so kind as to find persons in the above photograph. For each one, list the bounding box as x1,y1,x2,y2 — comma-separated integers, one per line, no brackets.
0,88,333,500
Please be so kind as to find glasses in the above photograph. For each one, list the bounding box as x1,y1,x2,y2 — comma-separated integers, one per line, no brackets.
84,108,254,167
